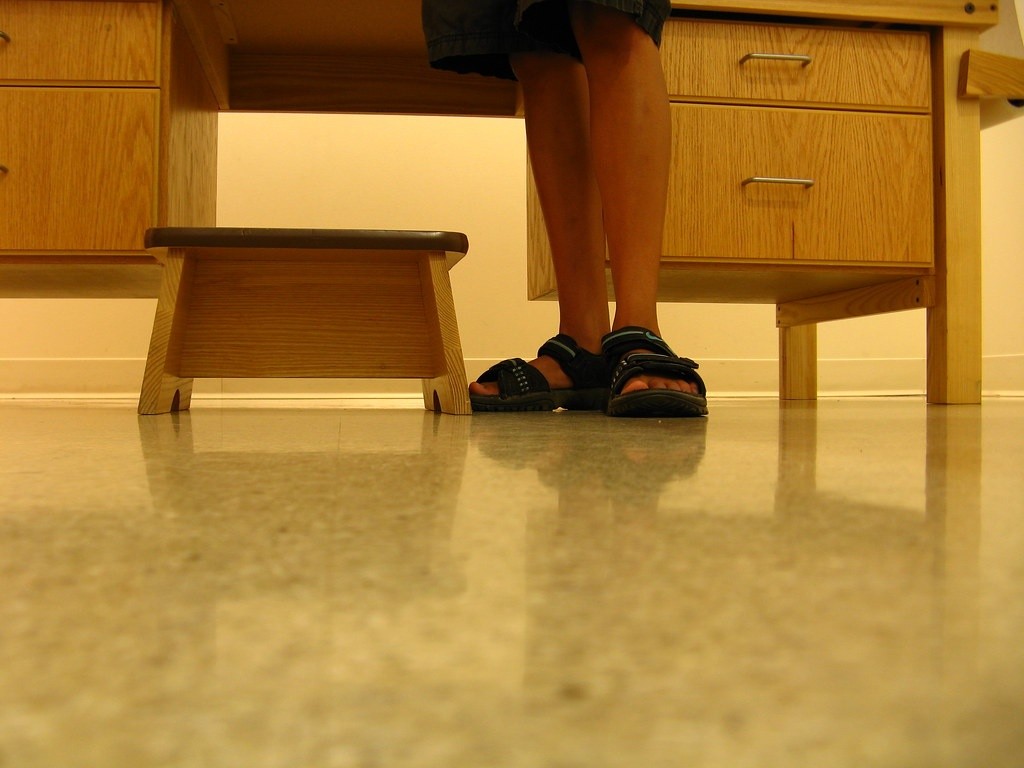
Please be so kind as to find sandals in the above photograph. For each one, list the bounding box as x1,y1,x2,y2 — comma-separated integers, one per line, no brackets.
601,326,709,415
469,334,610,411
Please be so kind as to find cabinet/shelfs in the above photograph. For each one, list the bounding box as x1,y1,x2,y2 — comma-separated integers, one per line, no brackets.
0,1,1002,405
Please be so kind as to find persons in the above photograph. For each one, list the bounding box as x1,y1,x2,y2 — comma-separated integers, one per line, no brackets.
421,0,708,417
469,416,705,520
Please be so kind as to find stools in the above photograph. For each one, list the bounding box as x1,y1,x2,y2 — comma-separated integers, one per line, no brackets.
136,227,472,418
